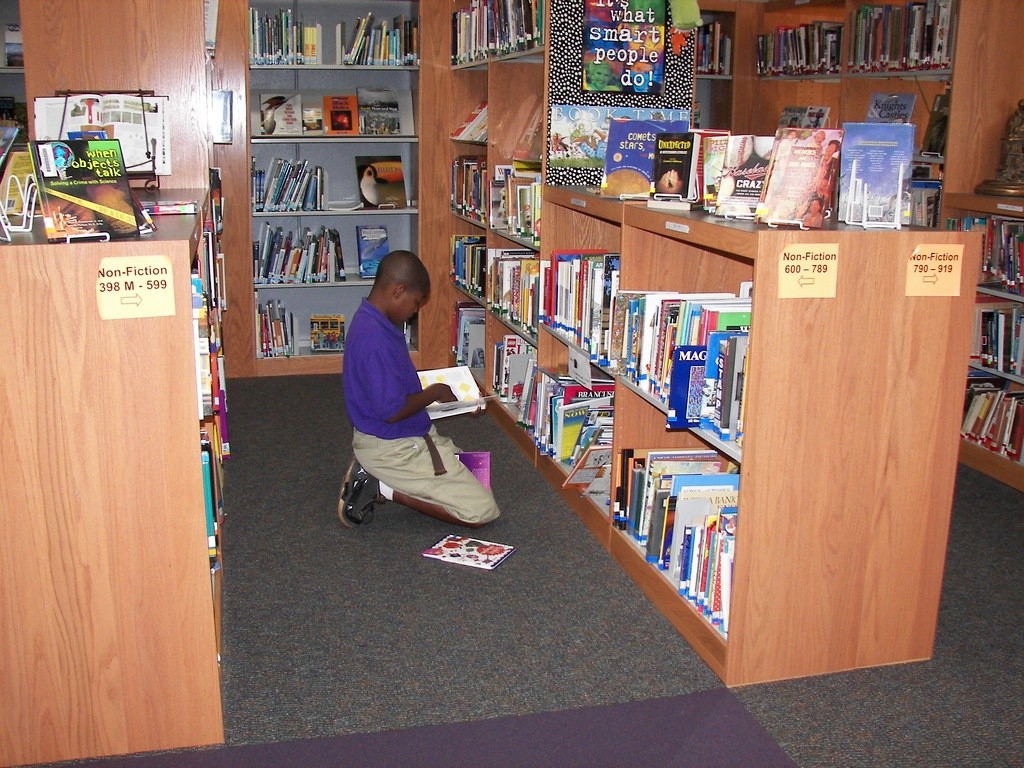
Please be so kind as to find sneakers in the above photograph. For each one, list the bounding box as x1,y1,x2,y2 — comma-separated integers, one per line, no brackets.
336,460,386,528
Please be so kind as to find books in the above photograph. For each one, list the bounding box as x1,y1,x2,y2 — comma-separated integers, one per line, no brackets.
0,23,196,243
417,366,497,420
945,214,1024,468
448,232,754,448
421,533,515,570
449,1,545,66
253,87,404,135
191,168,231,665
548,0,955,226
253,221,389,285
248,151,407,212
447,93,543,248
203,45,233,168
455,451,492,492
535,371,743,641
253,287,346,359
251,1,416,67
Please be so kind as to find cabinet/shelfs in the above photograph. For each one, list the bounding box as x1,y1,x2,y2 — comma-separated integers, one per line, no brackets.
0,0,1023,768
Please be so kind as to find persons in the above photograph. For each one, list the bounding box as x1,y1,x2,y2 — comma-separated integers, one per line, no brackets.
339,251,501,529
802,198,824,226
819,139,837,175
809,129,826,152
796,180,828,219
995,98,1024,185
